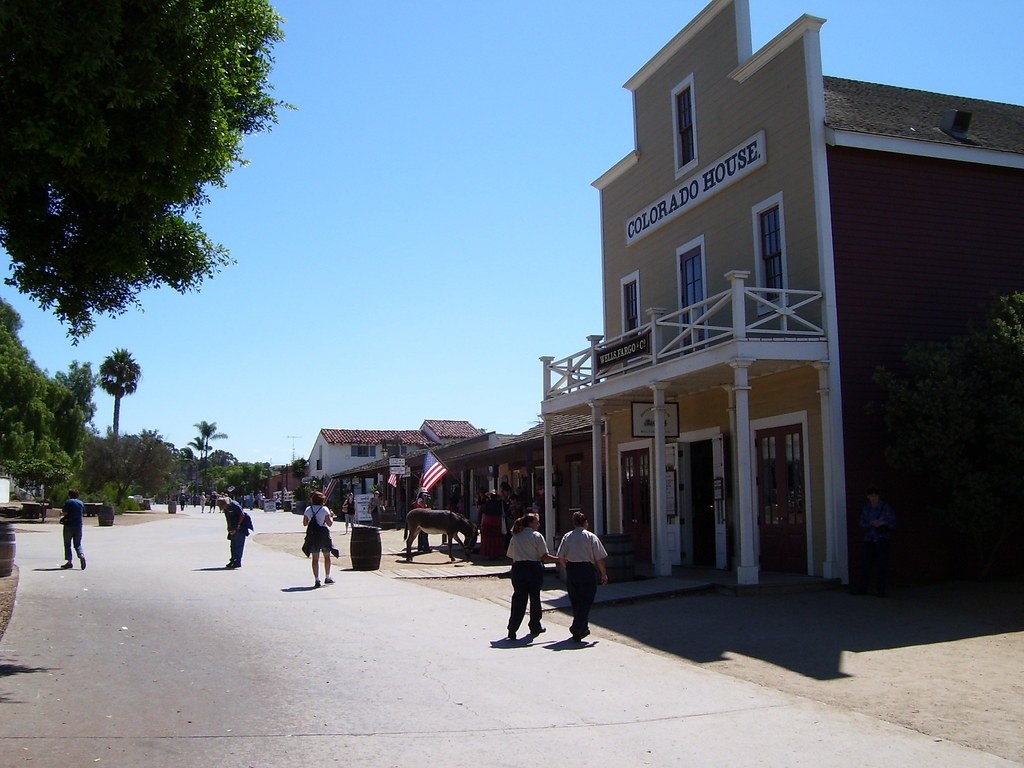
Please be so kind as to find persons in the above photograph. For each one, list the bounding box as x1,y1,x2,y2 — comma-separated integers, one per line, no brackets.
477,481,532,561
858,485,896,596
179,491,265,513
508,512,567,640
302,491,339,588
414,487,432,553
58,489,86,570
342,492,357,528
556,511,608,643
368,490,385,527
218,497,253,568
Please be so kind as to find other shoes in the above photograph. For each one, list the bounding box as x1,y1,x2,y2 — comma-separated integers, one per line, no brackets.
325,578,335,583
508,632,517,640
531,627,546,634
226,561,241,568
81,557,86,570
418,548,432,552
61,562,73,568
573,630,591,642
315,580,321,587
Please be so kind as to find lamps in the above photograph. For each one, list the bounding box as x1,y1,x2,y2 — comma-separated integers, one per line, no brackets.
939,107,972,141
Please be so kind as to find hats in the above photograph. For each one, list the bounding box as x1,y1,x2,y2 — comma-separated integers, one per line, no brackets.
419,487,428,493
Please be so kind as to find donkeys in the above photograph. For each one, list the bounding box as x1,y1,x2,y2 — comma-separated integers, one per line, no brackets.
404,508,478,562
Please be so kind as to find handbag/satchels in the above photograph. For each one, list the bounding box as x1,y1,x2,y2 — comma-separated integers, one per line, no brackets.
60,517,74,526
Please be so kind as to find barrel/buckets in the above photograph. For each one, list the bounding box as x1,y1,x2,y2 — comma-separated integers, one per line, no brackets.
169,501,176,514
0,522,16,577
598,535,634,581
350,527,381,571
379,505,396,530
99,506,114,526
284,501,292,512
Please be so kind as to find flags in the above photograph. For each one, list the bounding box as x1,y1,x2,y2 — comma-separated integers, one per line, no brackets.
412,449,448,504
387,473,397,487
